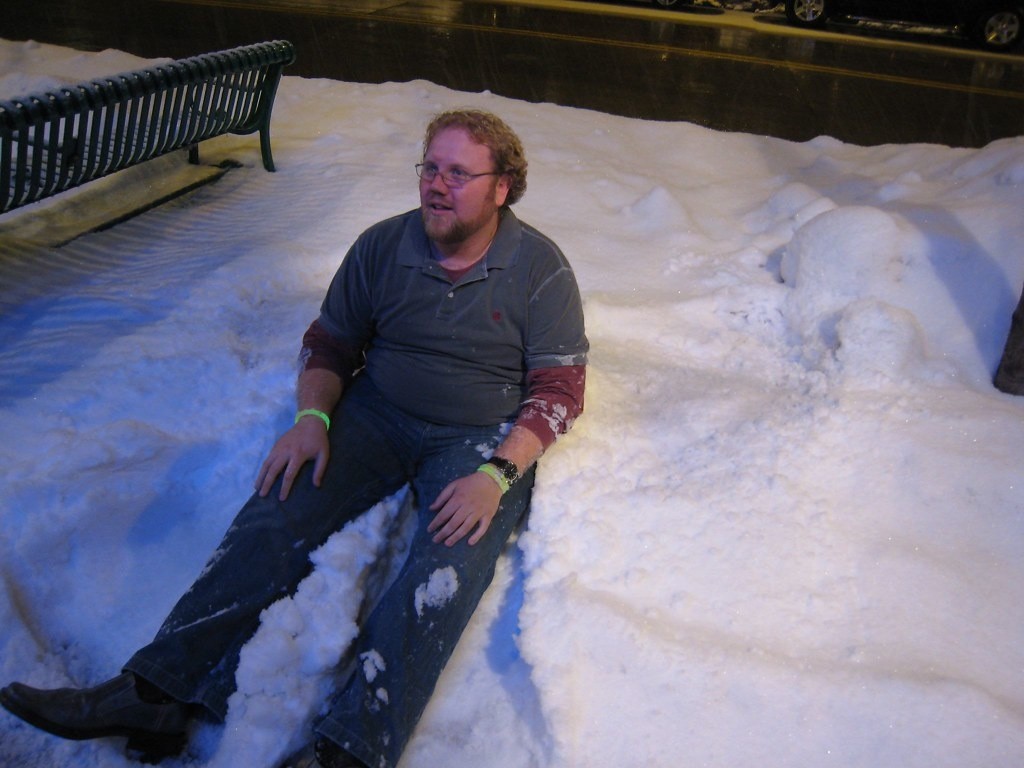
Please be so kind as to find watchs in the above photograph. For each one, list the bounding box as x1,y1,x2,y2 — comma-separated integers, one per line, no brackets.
487,456,519,487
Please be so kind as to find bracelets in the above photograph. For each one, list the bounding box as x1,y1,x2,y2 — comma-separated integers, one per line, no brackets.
295,409,330,431
477,464,509,494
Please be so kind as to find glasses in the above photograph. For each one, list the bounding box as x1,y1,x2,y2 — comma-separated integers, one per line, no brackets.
415,163,500,187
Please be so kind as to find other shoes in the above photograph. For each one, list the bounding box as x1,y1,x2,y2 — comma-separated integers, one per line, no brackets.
0,671,191,763
279,737,369,768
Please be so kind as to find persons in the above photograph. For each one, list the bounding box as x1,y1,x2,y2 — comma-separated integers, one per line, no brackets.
2,108,588,766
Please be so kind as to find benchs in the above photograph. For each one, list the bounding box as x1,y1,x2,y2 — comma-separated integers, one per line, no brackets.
0,38,296,214
